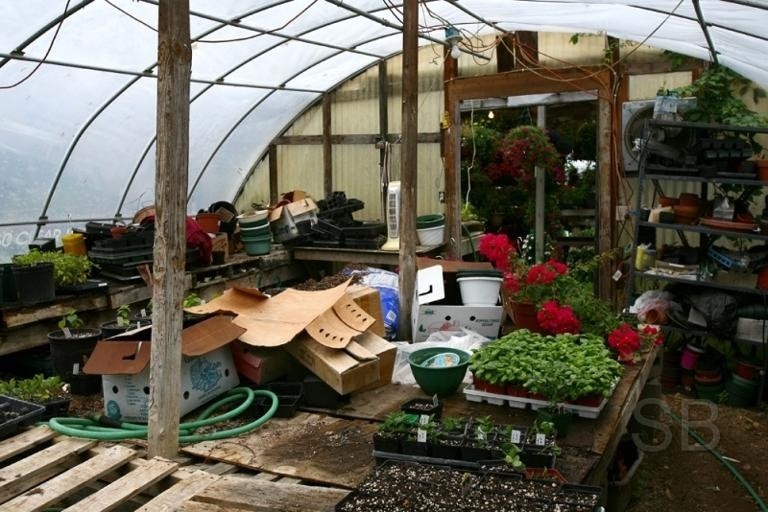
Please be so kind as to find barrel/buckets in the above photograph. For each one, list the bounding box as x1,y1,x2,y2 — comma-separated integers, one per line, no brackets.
12,262,57,303
0,263,17,303
100,318,151,336
135,311,152,319
47,328,103,396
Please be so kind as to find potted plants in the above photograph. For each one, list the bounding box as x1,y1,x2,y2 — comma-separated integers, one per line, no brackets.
371,329,619,482
1,246,103,422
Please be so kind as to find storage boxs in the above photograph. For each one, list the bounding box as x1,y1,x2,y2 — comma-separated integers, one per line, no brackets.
412,257,517,341
82,278,396,424
269,197,318,243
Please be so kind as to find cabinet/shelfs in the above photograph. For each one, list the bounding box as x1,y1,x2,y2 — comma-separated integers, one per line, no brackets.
627,120,767,342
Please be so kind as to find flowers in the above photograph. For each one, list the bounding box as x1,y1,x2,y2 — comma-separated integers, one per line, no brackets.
477,231,642,356
484,123,564,186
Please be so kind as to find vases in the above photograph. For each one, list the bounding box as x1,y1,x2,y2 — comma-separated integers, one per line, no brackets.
695,360,760,410
196,213,221,235
237,209,273,256
415,215,445,246
456,268,503,306
658,192,699,224
